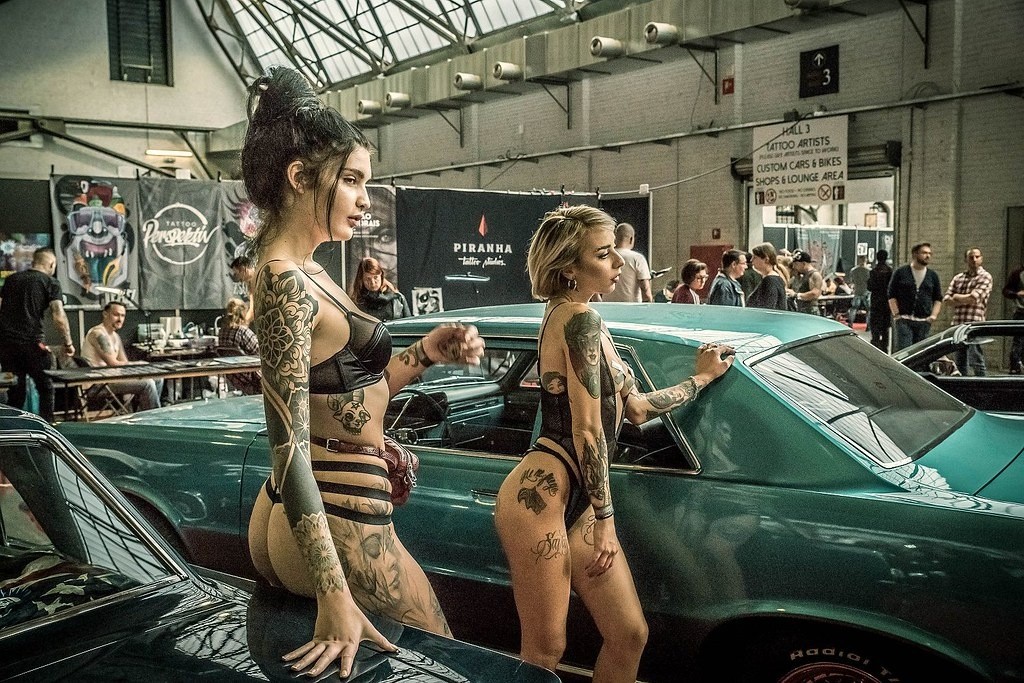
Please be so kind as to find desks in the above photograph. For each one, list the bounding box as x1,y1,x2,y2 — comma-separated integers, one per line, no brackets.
50,354,263,420
134,339,223,404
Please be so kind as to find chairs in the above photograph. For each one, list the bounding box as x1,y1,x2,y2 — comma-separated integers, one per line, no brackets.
71,355,135,420
213,347,264,396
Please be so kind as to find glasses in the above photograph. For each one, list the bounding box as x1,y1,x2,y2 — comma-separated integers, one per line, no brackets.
694,275,710,282
738,262,748,265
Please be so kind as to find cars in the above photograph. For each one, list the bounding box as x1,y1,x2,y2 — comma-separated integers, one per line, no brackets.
51,301,1024,683
0,403,560,682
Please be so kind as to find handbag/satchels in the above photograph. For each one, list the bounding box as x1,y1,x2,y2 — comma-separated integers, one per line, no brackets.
382,433,419,506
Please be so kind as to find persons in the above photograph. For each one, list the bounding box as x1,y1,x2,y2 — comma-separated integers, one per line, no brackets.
670,258,709,304
346,257,412,322
653,280,681,304
1001,250,1024,376
744,241,789,310
867,249,895,354
708,248,749,307
217,298,264,396
80,300,165,411
235,64,487,680
772,246,857,328
944,246,993,379
591,222,654,303
494,196,737,683
0,247,76,427
226,256,257,323
847,255,872,333
886,241,943,354
734,252,762,302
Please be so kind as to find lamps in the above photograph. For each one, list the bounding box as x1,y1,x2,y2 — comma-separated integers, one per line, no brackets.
454,71,482,91
590,36,625,60
644,23,680,44
490,61,519,81
356,101,383,113
384,92,411,107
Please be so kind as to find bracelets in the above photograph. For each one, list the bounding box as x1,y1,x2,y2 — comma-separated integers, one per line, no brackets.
63,343,71,348
687,375,698,401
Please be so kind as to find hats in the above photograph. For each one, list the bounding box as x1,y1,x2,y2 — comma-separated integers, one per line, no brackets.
792,252,812,263
